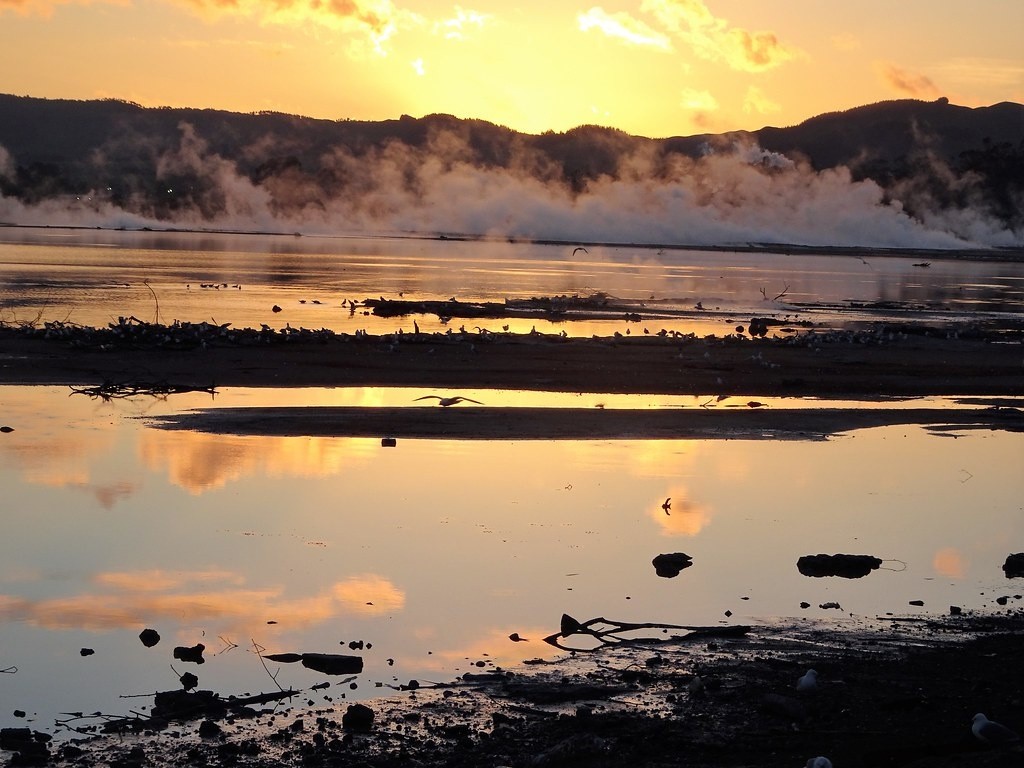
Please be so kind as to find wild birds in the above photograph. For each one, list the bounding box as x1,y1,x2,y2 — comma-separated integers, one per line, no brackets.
412,395,485,408
186,279,241,291
0,292,1024,388
572,248,588,256
